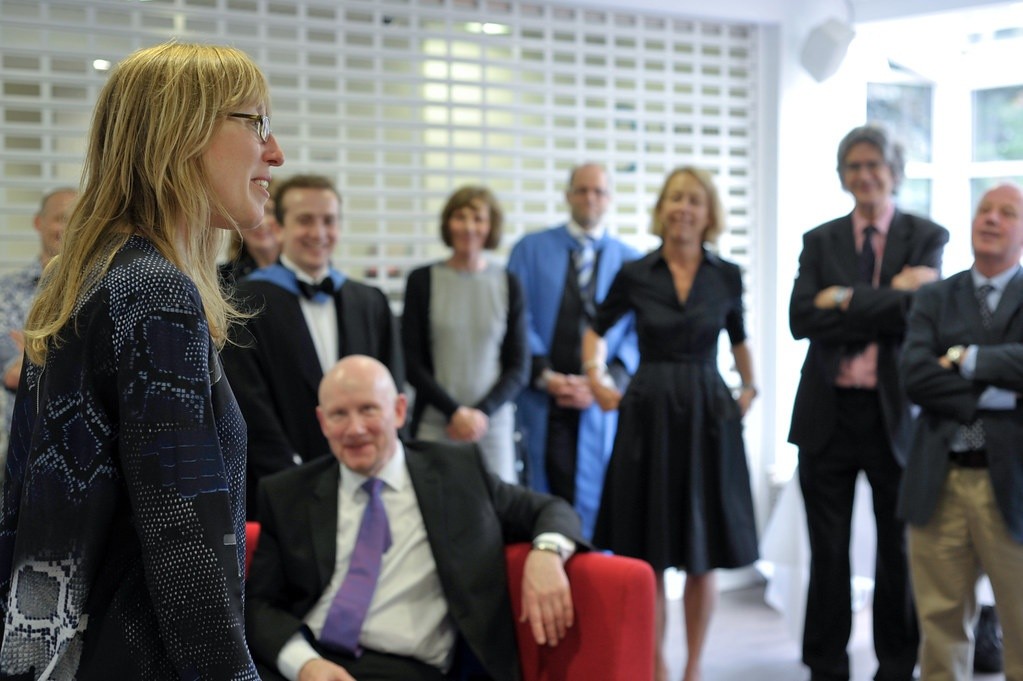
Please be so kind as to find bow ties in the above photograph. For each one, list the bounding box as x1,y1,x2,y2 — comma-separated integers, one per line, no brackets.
296,276,337,299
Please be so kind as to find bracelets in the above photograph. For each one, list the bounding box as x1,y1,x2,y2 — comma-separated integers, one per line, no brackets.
533,541,562,555
832,284,848,313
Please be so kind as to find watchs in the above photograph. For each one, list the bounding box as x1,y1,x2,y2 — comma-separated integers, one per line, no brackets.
946,344,963,370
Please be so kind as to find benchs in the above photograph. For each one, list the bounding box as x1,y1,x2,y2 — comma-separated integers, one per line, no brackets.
243,522,656,681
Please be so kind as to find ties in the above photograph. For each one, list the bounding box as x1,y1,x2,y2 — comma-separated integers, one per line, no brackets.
978,285,993,328
862,227,876,286
319,478,392,650
576,236,596,288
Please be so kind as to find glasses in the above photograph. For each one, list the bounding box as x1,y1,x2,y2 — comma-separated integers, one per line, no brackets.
228,112,270,142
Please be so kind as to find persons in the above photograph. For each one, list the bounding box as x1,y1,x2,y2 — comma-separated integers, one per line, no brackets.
240,354,587,680
574,163,756,681
215,166,410,504
888,181,1022,681
505,159,644,547
1,188,85,476
0,39,285,681
782,122,950,681
208,172,285,299
401,181,528,486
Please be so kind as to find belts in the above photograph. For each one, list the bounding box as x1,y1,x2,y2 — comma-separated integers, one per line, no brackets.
949,447,991,467
